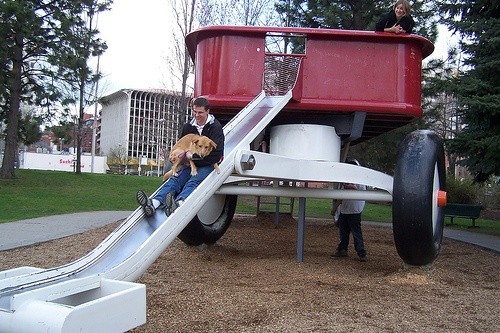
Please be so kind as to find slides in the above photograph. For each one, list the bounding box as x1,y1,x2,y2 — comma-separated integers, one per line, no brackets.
0,89,292,313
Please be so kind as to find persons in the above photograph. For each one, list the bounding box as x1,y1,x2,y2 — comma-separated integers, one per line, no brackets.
136,98,224,217
376,0,414,33
330,161,369,261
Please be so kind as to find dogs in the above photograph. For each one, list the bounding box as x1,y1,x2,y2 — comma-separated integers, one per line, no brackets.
169,133,221,177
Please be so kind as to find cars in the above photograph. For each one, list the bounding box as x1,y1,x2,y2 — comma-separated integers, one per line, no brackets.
122,169,139,176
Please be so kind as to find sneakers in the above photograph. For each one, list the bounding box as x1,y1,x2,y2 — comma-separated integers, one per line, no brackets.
164,192,178,217
360,255,369,261
331,250,348,257
136,190,156,216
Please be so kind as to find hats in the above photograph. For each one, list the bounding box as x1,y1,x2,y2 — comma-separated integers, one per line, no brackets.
348,158,360,166
